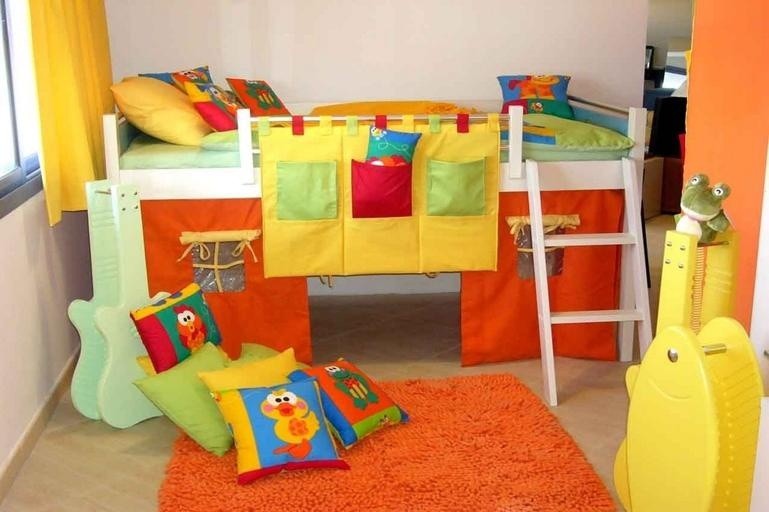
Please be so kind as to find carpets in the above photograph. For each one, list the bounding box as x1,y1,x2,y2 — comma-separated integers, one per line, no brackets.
158,373,620,512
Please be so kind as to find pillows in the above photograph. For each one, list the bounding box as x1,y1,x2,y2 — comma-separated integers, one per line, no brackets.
497,74,574,121
365,125,423,166
112,66,291,150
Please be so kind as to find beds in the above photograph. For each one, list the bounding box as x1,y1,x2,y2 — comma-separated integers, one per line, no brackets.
100,95,647,364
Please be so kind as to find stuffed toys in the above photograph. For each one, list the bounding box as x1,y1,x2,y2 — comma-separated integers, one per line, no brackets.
673,173,730,245
